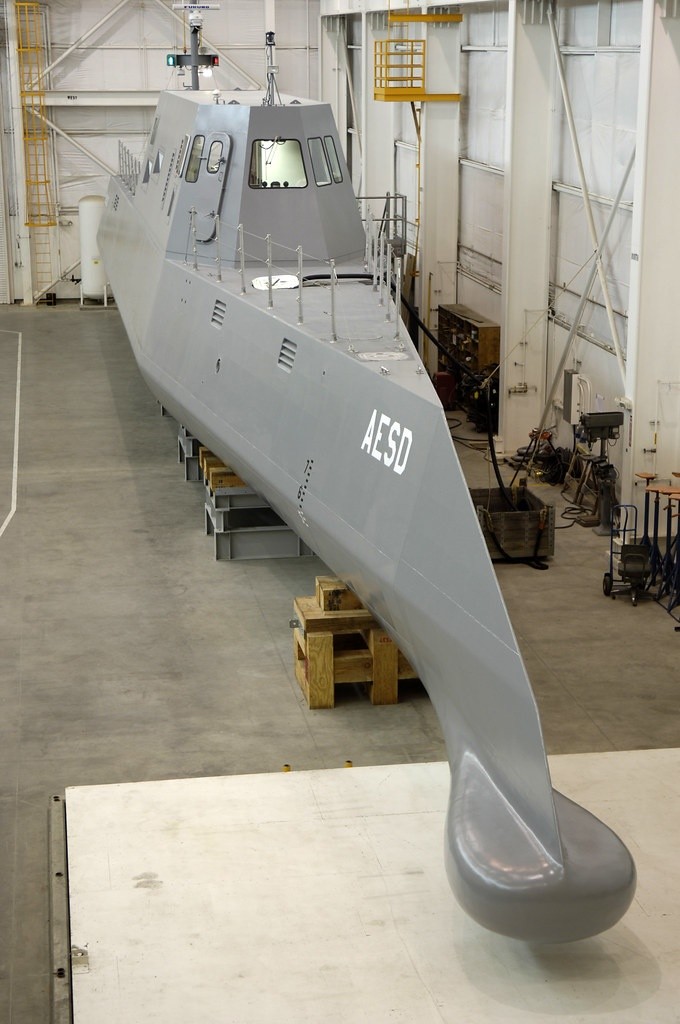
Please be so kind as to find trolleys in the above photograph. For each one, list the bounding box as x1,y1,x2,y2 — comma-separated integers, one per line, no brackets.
602,503,656,606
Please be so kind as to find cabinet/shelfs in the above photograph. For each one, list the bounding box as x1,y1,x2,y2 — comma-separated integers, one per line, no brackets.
437,303,500,372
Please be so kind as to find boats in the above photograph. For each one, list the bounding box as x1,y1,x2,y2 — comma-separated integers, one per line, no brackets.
97,1,639,959
